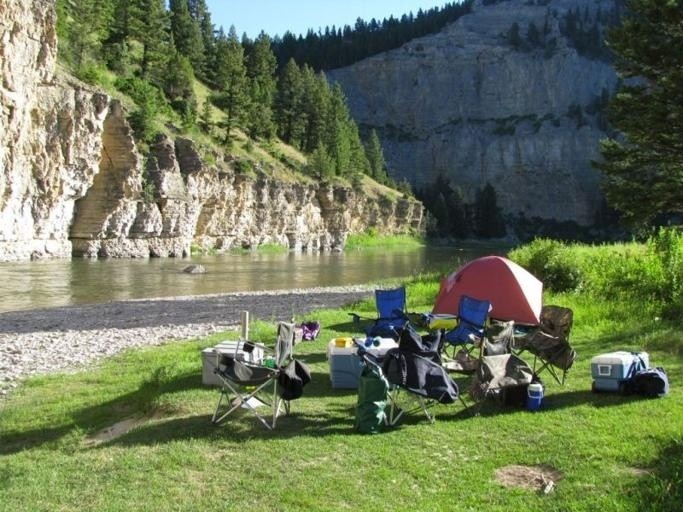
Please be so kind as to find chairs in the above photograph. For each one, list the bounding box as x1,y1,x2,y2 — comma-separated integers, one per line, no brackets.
352,318,446,425
473,319,531,416
207,320,297,432
443,294,493,362
514,303,574,386
363,285,408,337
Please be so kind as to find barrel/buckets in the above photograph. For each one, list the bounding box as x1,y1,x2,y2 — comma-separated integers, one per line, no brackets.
526,383,544,411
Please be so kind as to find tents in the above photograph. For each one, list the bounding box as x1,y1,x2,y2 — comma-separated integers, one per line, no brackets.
431,255,544,328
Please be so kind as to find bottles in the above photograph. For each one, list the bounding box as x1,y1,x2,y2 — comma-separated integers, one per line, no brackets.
373,335,382,347
364,336,373,347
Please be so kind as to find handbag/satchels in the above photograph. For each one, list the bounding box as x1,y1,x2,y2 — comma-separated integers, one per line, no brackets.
618,353,669,398
299,321,320,340
278,358,312,401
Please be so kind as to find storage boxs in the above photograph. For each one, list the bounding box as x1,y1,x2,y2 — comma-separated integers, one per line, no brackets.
324,336,400,390
590,350,650,393
201,348,252,386
213,339,265,366
